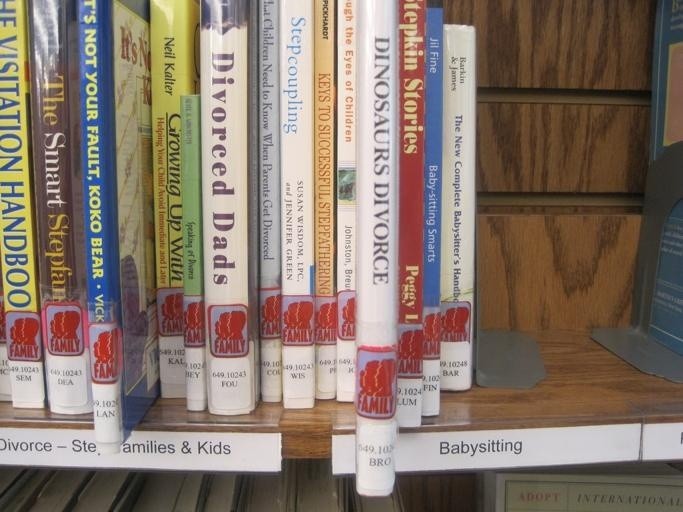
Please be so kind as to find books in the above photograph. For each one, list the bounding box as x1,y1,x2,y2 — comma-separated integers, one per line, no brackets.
630,3,682,355
3,458,682,511
1,1,476,497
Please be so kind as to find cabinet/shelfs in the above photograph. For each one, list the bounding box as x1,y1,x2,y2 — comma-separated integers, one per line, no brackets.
2,1,682,511
